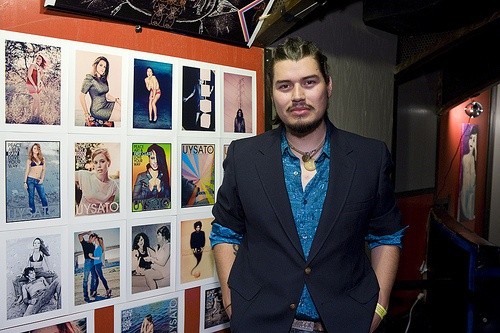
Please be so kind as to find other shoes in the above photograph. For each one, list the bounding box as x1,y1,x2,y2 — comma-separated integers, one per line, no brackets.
84,296,89,300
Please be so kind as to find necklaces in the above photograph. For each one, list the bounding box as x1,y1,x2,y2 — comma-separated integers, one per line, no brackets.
287,136,324,171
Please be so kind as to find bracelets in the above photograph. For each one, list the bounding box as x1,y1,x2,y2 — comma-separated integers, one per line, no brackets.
225,304,231,311
375,302,387,319
24,182,26,183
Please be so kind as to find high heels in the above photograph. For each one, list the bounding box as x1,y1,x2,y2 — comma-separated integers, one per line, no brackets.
92,291,97,297
104,289,112,296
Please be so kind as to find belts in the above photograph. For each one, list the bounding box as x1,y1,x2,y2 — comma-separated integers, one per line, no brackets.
292,318,325,332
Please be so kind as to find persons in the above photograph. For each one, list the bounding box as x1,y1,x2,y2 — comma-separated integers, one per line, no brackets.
24,143,49,216
132,225,170,290
11,237,61,317
79,56,121,127
78,231,112,302
27,55,46,123
184,79,213,122
211,296,222,318
209,37,410,333
182,176,200,204
234,109,246,133
141,314,153,333
132,144,171,211
190,221,205,252
75,148,117,214
144,67,161,124
